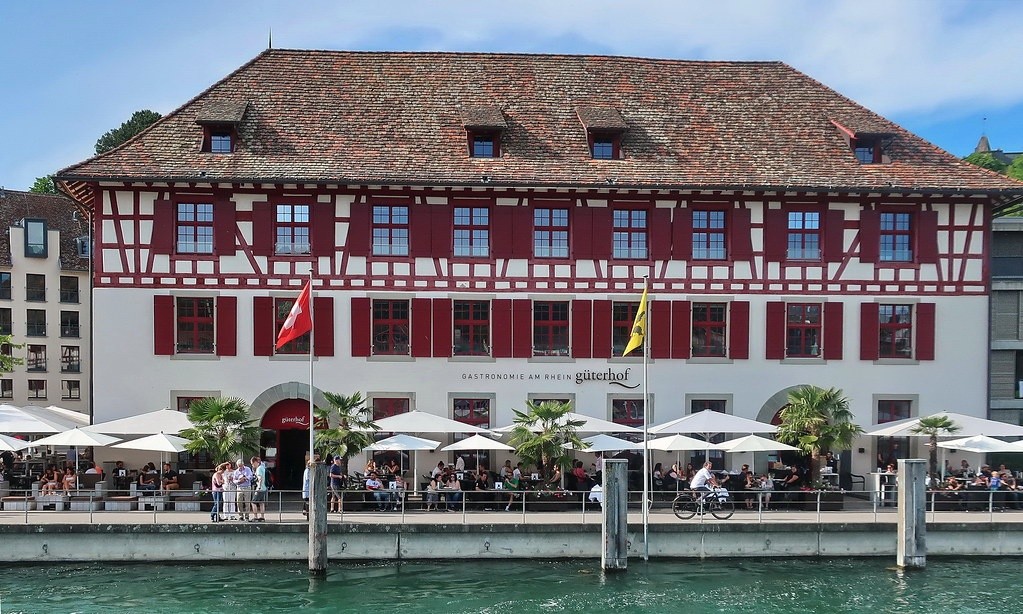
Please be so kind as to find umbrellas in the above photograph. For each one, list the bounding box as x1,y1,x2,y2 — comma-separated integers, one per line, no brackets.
0,403,271,496
716,433,803,475
860,410,1023,482
634,434,729,474
636,409,795,464
336,408,515,497
560,434,643,457
489,411,644,489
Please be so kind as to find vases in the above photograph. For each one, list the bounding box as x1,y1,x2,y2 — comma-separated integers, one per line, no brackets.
527,495,578,510
799,492,844,511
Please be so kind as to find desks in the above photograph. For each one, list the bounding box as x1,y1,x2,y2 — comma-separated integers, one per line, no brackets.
138,496,166,511
36,496,69,511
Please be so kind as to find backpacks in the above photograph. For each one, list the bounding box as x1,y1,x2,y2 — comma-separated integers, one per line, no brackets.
265,467,275,488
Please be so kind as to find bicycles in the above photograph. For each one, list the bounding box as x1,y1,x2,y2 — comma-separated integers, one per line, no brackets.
673,487,735,520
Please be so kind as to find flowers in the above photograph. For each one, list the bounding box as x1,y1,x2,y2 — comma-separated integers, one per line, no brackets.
800,480,833,493
521,482,572,499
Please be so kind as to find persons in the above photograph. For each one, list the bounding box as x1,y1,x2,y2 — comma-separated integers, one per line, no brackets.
925,458,1023,512
499,460,526,511
827,452,838,473
885,463,898,486
653,463,698,500
738,464,801,509
690,461,719,515
302,455,487,522
536,464,562,496
0,446,267,524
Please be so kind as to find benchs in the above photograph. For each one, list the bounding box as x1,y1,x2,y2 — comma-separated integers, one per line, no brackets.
1,473,203,511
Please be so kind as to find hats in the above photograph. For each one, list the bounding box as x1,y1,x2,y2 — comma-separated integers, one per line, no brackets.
334,456,343,460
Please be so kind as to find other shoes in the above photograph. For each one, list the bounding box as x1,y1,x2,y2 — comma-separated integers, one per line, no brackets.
68,492,71,497
337,511,345,514
696,499,701,506
330,510,336,513
211,514,265,522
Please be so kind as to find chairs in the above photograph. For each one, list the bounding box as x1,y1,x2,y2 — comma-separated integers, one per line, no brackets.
959,483,1007,512
364,480,495,512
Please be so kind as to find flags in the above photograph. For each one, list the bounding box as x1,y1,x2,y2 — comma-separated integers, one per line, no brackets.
276,280,312,350
622,286,647,358
